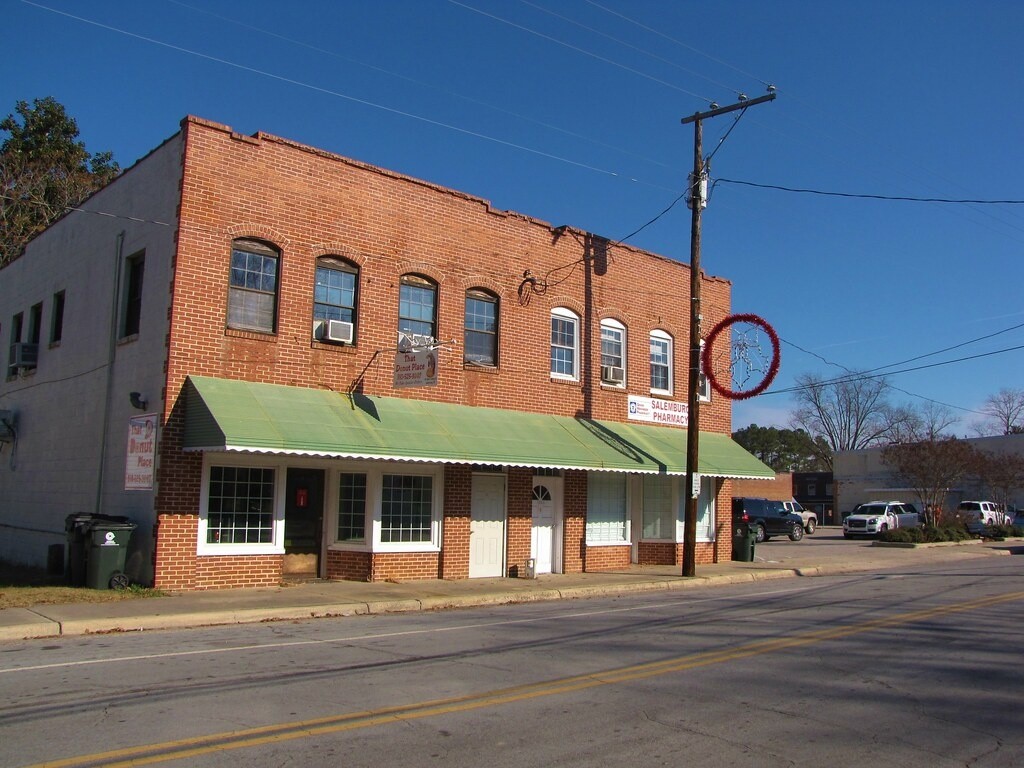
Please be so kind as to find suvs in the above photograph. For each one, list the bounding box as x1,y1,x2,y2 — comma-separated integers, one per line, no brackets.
733,497,805,543
842,500,920,540
954,500,1012,535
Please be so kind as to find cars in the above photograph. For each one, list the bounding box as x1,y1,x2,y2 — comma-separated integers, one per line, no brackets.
1010,509,1024,536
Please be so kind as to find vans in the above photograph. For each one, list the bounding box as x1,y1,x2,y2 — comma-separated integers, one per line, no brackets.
771,496,818,535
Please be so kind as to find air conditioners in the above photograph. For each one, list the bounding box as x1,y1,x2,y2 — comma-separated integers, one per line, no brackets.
409,333,435,353
324,318,353,344
9,342,37,368
0,408,14,438
603,365,624,382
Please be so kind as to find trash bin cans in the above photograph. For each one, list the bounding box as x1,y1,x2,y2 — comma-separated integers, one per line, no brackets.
65,512,139,591
732,521,759,562
